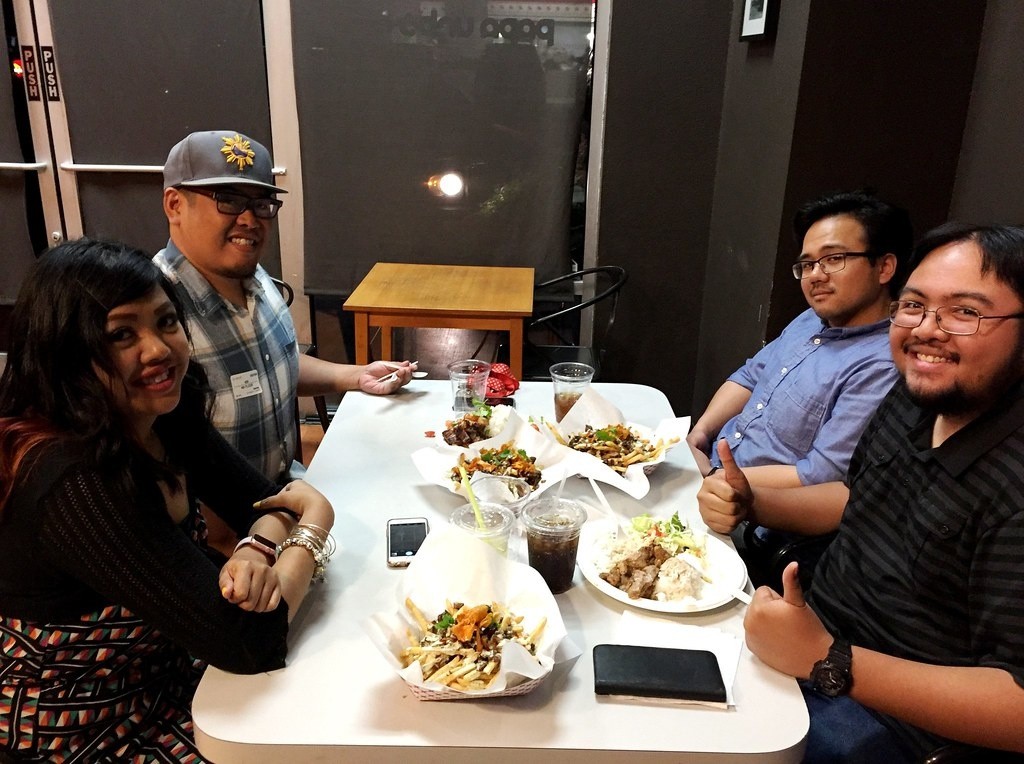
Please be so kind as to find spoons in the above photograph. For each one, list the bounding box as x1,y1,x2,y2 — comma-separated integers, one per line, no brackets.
380,362,428,377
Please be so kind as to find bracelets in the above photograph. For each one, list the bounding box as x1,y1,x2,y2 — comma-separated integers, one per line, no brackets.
274,523,336,584
706,465,720,477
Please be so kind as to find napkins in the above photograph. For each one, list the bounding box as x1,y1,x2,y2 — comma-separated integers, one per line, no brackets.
547,384,693,502
408,407,580,504
607,605,744,713
356,519,568,698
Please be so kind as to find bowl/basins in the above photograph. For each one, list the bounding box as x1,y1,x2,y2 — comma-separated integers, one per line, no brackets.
468,476,530,520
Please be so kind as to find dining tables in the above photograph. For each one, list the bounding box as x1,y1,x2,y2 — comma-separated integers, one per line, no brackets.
190,380,811,764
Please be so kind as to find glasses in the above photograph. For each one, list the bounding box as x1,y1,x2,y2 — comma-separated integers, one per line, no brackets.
174,182,284,220
791,252,871,278
888,298,1023,335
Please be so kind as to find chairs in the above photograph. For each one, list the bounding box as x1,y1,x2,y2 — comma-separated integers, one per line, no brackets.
470,264,628,381
270,276,355,434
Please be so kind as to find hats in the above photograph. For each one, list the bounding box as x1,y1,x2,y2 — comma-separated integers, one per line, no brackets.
163,131,289,194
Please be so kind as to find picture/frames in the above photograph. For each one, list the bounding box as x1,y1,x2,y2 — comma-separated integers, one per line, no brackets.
738,0,782,43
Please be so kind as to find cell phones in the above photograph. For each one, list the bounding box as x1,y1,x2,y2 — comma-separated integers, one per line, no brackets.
386,517,430,568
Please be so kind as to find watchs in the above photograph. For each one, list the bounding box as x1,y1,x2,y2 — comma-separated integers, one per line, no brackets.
808,637,854,702
232,533,280,566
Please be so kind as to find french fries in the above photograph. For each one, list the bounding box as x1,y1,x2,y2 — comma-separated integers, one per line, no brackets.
451,438,544,492
547,422,681,473
402,597,548,689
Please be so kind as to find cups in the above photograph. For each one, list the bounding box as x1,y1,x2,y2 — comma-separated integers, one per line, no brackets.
453,502,517,560
447,359,491,413
520,496,588,596
548,362,595,425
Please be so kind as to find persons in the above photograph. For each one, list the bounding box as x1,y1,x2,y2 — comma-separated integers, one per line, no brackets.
697,217,1024,764
150,129,418,491
685,194,903,598
0,235,334,764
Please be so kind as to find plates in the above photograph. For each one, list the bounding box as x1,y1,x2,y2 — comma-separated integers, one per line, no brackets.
570,510,748,613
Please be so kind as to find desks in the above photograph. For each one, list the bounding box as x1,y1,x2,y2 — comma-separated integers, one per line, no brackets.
341,262,536,381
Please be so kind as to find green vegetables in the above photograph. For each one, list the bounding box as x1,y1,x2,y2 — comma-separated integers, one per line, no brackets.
632,512,686,541
471,398,619,462
